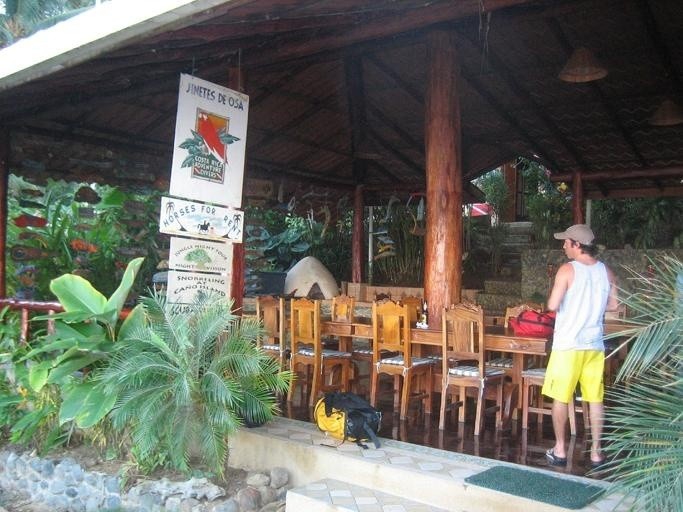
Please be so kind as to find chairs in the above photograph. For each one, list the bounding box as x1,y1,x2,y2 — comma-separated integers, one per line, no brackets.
250,290,630,439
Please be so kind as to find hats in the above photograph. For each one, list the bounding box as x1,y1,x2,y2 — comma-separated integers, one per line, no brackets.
553,224,594,247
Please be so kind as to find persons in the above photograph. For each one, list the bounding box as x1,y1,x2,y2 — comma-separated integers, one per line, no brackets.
539,222,618,467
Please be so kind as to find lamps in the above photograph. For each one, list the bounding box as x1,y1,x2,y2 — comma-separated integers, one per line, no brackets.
639,88,683,129
553,30,614,87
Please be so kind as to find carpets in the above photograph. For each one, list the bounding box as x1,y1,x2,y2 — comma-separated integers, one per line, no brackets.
462,464,608,512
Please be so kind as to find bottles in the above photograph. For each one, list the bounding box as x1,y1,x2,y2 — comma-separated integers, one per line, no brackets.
421,300,428,324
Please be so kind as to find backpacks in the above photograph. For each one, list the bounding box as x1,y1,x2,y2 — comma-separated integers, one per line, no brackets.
313,392,382,451
508,309,555,338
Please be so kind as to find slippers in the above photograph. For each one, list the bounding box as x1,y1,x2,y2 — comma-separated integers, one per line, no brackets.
591,452,606,466
546,449,567,464
